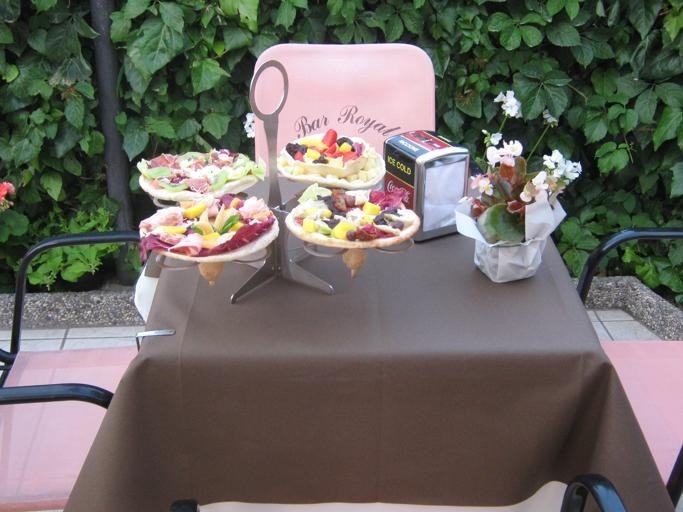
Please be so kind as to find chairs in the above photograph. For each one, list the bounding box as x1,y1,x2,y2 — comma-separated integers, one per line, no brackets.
246,42,435,176
0,229,146,512
168,476,624,512
577,228,683,512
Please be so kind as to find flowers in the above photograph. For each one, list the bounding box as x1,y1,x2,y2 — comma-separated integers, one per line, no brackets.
467,89,584,243
